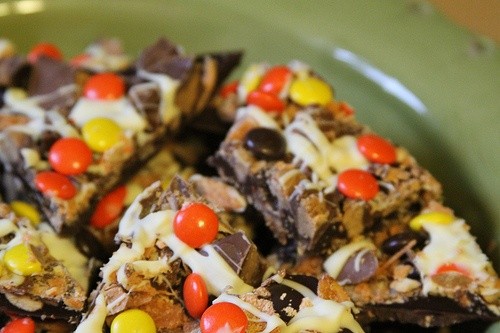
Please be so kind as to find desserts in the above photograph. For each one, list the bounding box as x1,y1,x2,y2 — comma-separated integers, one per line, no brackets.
0,36,500,332
